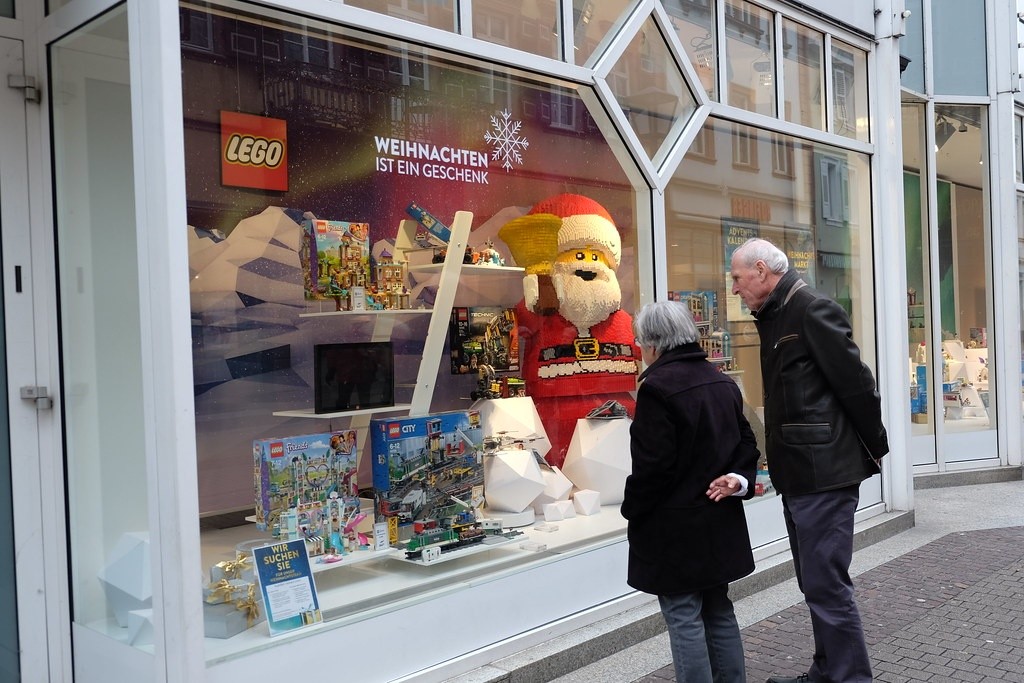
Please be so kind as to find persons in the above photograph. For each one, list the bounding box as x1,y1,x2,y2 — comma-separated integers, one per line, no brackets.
730,238,890,683
621,299,760,683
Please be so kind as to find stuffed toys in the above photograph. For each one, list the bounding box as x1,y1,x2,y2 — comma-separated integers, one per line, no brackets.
515,194,643,471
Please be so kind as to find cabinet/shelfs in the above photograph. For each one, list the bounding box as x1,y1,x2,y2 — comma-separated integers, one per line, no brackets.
244,211,533,575
909,304,925,344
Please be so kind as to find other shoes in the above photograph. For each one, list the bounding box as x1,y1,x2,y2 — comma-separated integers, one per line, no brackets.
766,672,809,683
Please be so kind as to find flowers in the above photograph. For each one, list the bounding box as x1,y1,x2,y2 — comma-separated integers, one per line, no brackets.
979,357,988,368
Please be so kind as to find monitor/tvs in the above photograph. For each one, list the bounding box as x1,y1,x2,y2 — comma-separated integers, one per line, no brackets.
313,341,395,413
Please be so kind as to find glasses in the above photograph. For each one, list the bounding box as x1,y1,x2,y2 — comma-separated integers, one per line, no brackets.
634,337,641,348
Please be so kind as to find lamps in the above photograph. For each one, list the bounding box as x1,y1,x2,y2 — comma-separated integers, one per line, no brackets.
935,113,956,153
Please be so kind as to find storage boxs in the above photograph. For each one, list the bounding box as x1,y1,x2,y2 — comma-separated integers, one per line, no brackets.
673,291,718,333
253,430,360,532
301,219,370,301
910,364,959,424
970,327,987,348
406,202,451,243
370,409,484,527
450,306,520,374
203,553,267,639
